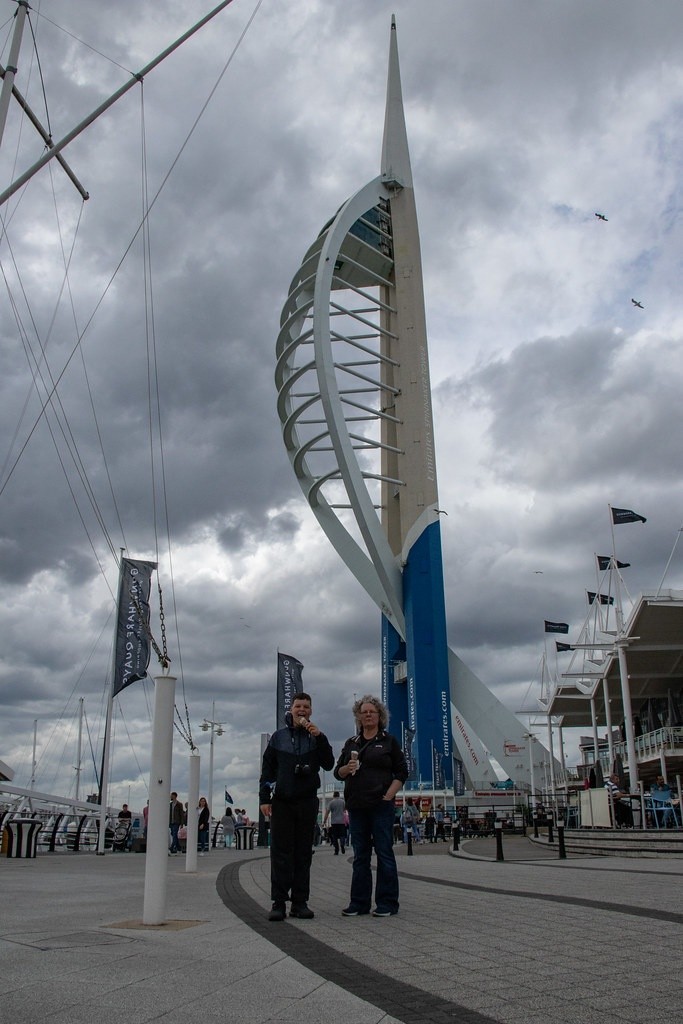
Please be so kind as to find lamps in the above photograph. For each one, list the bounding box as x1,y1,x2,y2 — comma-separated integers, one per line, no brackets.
596,717,599,720
609,699,613,703
628,674,632,679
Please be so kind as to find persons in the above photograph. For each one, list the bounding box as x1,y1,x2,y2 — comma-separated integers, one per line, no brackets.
118,791,489,855
604,772,676,830
259,693,335,921
0,808,14,828
333,695,409,916
507,801,545,829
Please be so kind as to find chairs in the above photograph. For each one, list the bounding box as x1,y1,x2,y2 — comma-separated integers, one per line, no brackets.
565,789,680,830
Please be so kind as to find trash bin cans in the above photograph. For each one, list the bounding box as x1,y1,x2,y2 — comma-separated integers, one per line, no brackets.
234,827,256,851
6,819,43,858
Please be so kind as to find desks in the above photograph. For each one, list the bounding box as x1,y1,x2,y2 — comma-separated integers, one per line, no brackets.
622,795,651,829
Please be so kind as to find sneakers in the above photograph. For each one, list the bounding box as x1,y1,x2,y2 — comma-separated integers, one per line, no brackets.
269,902,287,921
288,903,314,919
342,904,370,915
373,907,398,916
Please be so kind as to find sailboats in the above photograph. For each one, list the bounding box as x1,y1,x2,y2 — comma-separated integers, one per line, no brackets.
10,696,115,852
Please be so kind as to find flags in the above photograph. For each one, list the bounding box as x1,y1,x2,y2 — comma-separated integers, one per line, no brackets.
276,653,304,730
225,791,233,804
433,746,446,790
556,642,576,652
588,591,614,605
454,757,466,796
611,508,646,525
597,556,630,570
404,725,416,785
112,558,158,698
545,621,569,634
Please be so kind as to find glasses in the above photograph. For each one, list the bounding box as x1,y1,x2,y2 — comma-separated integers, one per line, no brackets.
360,710,379,714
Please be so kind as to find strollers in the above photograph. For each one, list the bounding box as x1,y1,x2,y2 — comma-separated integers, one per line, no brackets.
111,820,133,852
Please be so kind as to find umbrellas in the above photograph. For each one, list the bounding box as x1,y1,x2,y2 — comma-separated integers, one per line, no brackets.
619,714,644,744
648,697,662,732
668,688,682,733
584,760,604,790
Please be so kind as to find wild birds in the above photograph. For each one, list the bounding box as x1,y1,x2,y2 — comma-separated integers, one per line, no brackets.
632,298,643,309
595,213,608,221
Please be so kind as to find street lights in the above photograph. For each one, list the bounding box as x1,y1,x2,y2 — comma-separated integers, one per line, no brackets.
198,699,227,853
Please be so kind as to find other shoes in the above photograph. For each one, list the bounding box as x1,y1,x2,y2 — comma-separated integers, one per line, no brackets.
168,849,178,856
443,839,449,842
200,851,205,856
434,840,438,843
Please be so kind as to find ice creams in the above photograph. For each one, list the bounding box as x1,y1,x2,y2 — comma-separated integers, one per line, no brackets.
351,751,358,775
299,717,308,726
